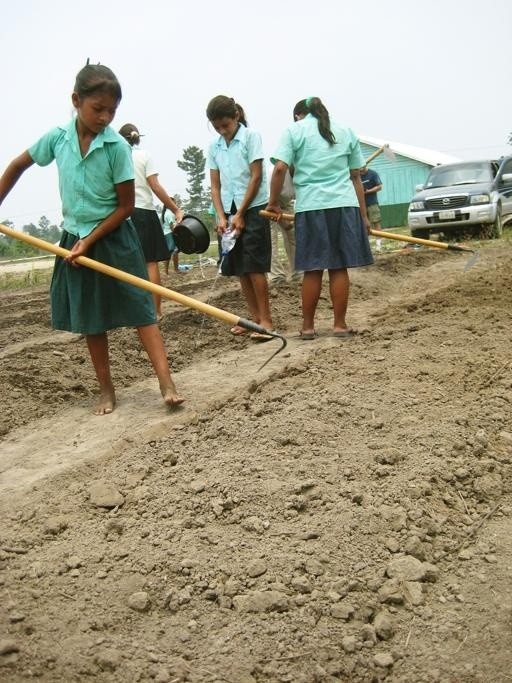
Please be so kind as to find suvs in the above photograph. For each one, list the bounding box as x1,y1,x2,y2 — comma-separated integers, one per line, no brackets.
407,152,512,239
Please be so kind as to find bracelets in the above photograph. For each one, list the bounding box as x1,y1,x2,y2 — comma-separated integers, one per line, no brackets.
172,206,179,213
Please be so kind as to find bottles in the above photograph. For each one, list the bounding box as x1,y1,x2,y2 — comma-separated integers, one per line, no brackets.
179,264,195,272
219,212,241,255
415,242,421,250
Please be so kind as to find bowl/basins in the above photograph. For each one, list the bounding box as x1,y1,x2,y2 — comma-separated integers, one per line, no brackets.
172,214,211,255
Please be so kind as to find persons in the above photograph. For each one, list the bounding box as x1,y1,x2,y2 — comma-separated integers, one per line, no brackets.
1,62,188,416
266,161,303,286
204,94,280,339
159,194,182,275
265,94,372,340
210,199,225,274
350,166,385,255
117,121,187,322
